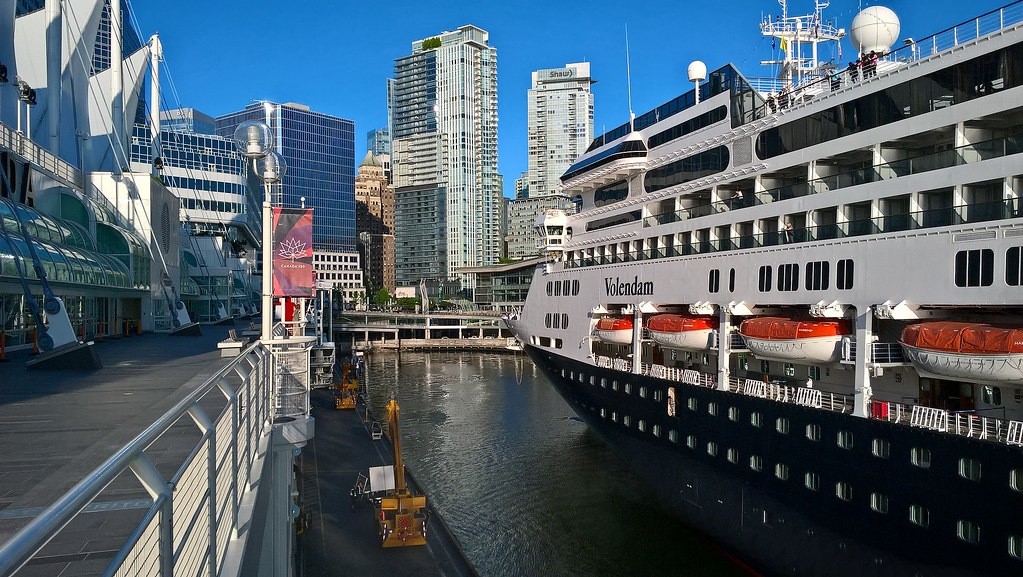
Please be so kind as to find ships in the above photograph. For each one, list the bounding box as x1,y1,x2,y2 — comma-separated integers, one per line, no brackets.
506,0,1023,577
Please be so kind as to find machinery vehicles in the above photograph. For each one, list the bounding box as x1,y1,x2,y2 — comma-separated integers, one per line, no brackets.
332,362,360,410
345,390,432,549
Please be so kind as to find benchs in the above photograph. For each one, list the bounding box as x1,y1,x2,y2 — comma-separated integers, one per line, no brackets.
217,337,251,358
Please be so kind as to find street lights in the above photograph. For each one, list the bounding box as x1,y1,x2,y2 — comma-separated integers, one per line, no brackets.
231,118,288,342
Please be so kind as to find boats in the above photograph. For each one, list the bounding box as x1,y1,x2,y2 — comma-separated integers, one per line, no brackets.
643,313,721,354
732,314,859,368
894,320,1023,386
595,318,645,346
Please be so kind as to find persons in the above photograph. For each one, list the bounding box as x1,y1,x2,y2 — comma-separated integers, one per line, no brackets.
766,93,776,114
824,67,842,93
685,356,693,370
778,84,796,112
846,61,859,84
805,375,813,389
731,189,744,208
856,50,878,81
